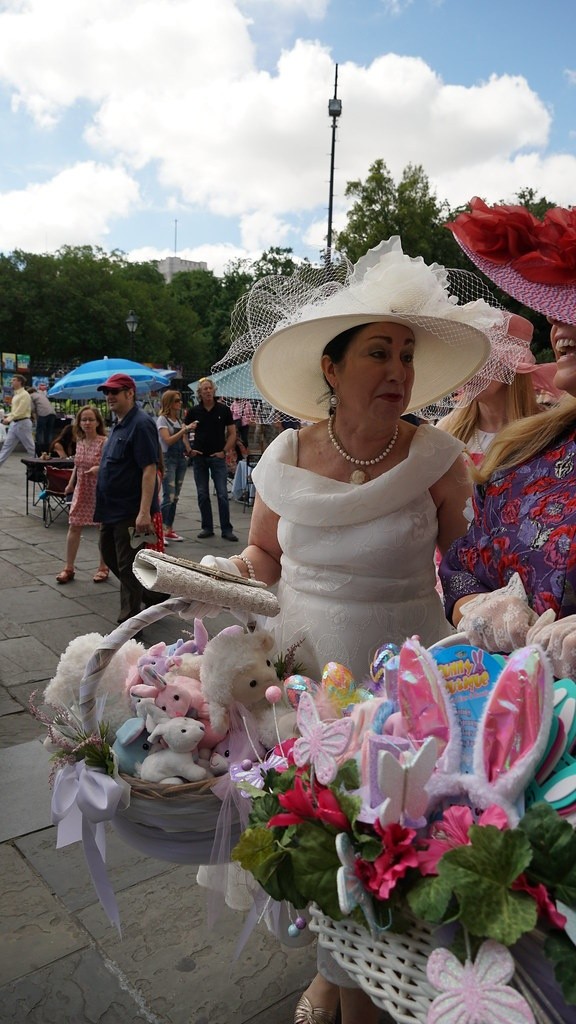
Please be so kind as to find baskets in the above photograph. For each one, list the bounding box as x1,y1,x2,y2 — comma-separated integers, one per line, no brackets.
79,597,278,866
309,631,576,1024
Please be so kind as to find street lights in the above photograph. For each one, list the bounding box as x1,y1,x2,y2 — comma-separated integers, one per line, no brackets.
125,310,139,360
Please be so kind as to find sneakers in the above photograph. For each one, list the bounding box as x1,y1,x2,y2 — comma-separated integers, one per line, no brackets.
165,529,183,541
164,537,169,545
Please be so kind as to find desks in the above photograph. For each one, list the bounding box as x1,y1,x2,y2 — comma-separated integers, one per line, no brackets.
232,460,259,505
21,458,76,514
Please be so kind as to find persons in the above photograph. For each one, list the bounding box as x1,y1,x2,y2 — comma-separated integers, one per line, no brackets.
182,377,238,542
136,389,161,416
0,375,35,466
27,387,57,457
50,425,76,458
157,389,198,545
433,308,566,603
217,396,302,479
437,194,576,682
178,235,530,1024
56,404,110,583
93,374,171,623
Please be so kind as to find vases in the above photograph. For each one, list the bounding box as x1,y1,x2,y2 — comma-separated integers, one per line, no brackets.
309,887,470,1024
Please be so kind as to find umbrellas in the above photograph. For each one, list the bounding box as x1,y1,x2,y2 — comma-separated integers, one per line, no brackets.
188,358,262,399
46,354,170,400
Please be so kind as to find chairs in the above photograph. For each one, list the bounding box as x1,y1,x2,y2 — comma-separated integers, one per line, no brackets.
40,466,75,528
239,454,261,515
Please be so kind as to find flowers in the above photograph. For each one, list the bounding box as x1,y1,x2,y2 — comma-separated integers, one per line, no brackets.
233,698,576,1003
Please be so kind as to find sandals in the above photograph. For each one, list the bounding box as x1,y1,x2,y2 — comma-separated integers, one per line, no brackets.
93,569,109,583
293,994,337,1024
56,569,75,582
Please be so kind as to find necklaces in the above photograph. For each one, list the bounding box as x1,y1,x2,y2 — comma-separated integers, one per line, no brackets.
327,412,398,483
474,425,486,456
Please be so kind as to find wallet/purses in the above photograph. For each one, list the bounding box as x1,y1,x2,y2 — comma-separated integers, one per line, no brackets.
131,549,280,617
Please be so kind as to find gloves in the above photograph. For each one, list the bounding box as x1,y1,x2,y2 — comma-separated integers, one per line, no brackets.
179,555,241,620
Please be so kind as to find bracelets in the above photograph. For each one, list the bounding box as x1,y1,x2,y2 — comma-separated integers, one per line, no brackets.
229,555,256,580
223,450,227,455
189,450,192,454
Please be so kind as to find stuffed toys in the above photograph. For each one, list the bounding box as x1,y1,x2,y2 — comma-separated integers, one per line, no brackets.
398,640,553,827
44,618,298,784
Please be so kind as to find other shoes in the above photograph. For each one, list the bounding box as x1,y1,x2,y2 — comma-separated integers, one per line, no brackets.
222,530,238,541
197,528,213,539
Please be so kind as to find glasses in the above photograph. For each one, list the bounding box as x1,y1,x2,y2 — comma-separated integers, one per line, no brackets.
103,387,128,396
175,398,183,403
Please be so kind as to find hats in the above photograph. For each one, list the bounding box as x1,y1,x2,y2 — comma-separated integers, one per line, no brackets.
212,235,532,425
443,197,576,327
96,374,136,391
494,310,567,400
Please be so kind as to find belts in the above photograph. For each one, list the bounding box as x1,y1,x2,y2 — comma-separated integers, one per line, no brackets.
12,417,28,423
